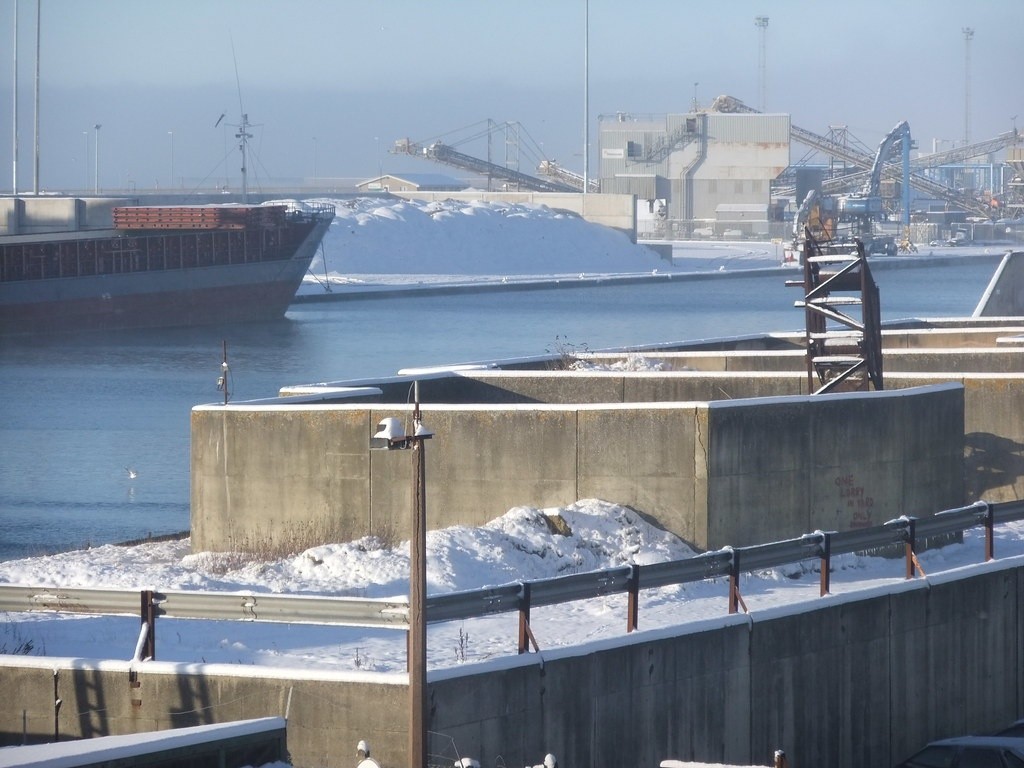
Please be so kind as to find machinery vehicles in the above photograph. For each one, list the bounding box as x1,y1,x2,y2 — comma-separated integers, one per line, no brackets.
808,121,918,257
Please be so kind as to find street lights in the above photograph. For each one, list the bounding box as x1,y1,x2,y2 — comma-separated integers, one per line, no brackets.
168,131,173,189
755,16,771,117
93,124,101,195
962,27,976,162
83,132,89,188
375,137,382,185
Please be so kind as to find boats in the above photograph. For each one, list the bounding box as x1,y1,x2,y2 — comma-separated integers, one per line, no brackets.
0,196,337,330
